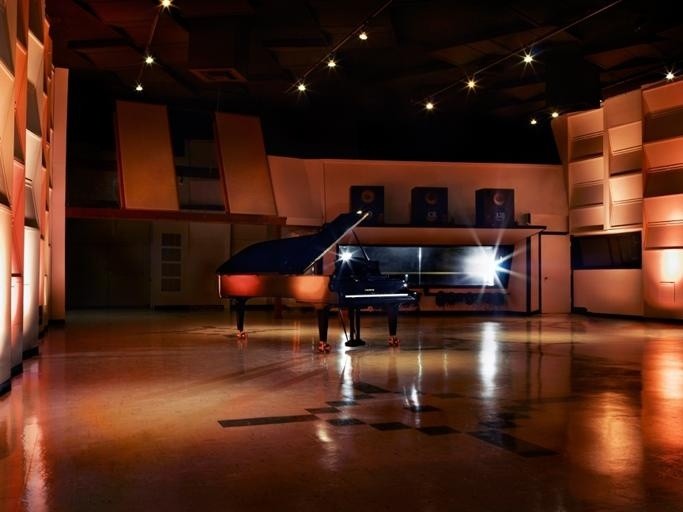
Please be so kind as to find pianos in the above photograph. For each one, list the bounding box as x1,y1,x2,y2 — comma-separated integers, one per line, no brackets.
216,210,421,353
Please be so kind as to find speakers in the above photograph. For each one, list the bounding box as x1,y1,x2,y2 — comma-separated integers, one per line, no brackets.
409,184,450,226
349,184,387,226
474,187,517,228
